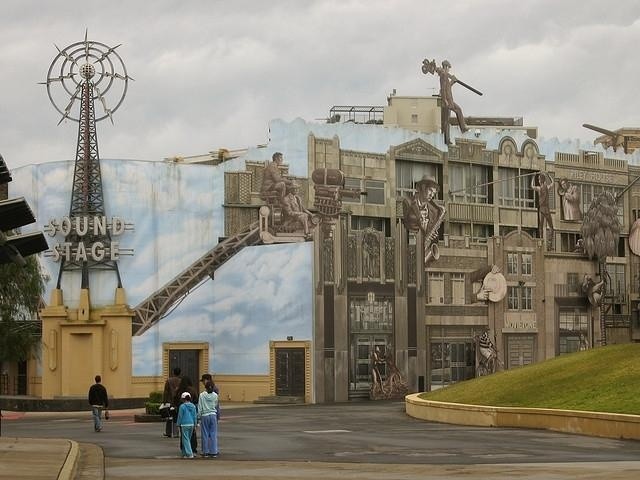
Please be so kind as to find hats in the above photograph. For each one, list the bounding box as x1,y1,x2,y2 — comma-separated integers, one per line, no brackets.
199,374,211,381
181,392,191,398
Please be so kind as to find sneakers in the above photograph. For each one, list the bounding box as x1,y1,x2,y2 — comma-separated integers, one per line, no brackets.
183,452,196,458
200,454,217,458
96,426,102,432
163,432,171,437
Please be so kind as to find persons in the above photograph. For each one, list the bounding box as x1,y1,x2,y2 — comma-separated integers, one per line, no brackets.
582,275,605,306
88,375,109,433
162,367,182,439
383,342,405,396
175,391,198,460
259,151,292,208
435,58,470,146
556,175,573,223
197,380,221,459
281,180,315,239
199,373,222,425
530,169,556,239
370,345,387,397
173,376,200,457
405,174,442,243
564,181,584,221
576,331,589,353
469,262,502,304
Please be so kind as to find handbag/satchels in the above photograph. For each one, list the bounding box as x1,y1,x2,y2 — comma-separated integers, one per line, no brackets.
159,403,174,421
104,412,109,420
